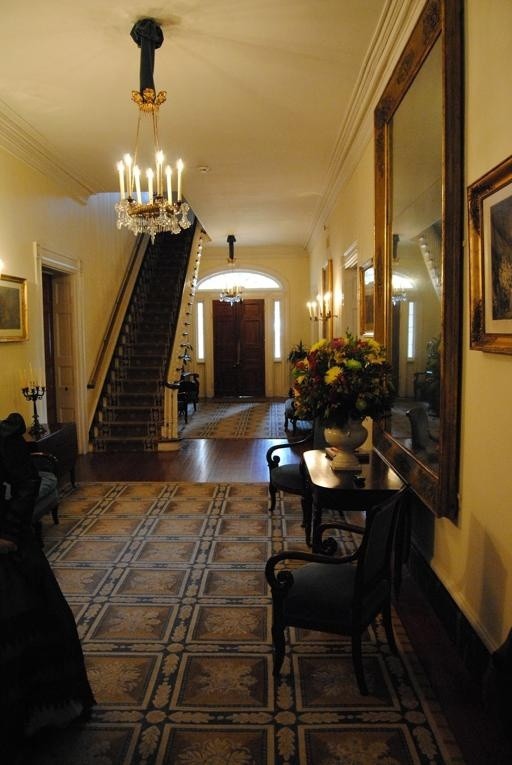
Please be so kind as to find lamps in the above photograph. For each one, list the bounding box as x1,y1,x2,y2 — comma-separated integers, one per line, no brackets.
112,16,195,245
216,235,246,308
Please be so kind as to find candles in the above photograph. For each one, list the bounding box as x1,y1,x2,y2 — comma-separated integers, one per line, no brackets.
305,291,331,322
17,362,46,390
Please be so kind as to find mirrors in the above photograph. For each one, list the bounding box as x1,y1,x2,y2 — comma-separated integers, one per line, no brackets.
319,257,336,343
357,257,376,338
372,1,466,529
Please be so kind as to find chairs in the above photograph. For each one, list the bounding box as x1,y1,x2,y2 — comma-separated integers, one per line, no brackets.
0,409,65,545
177,364,202,424
263,397,350,533
404,401,441,469
265,485,420,696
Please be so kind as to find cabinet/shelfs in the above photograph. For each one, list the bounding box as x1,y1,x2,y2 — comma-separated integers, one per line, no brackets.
21,421,78,489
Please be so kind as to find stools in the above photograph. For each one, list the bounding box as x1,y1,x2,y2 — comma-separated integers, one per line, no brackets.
285,398,314,436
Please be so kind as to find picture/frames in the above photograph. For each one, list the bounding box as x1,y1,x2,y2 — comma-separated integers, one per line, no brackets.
462,148,512,359
0,274,32,342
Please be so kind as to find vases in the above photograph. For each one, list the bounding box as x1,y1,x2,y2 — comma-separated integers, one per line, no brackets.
323,416,368,472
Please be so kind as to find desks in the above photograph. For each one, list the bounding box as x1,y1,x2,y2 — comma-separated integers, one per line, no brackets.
298,446,418,617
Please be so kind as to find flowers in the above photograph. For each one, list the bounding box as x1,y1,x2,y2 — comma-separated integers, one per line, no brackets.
287,329,402,429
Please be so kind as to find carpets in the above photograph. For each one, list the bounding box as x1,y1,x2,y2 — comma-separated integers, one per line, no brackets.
0,481,466,763
176,399,313,440
